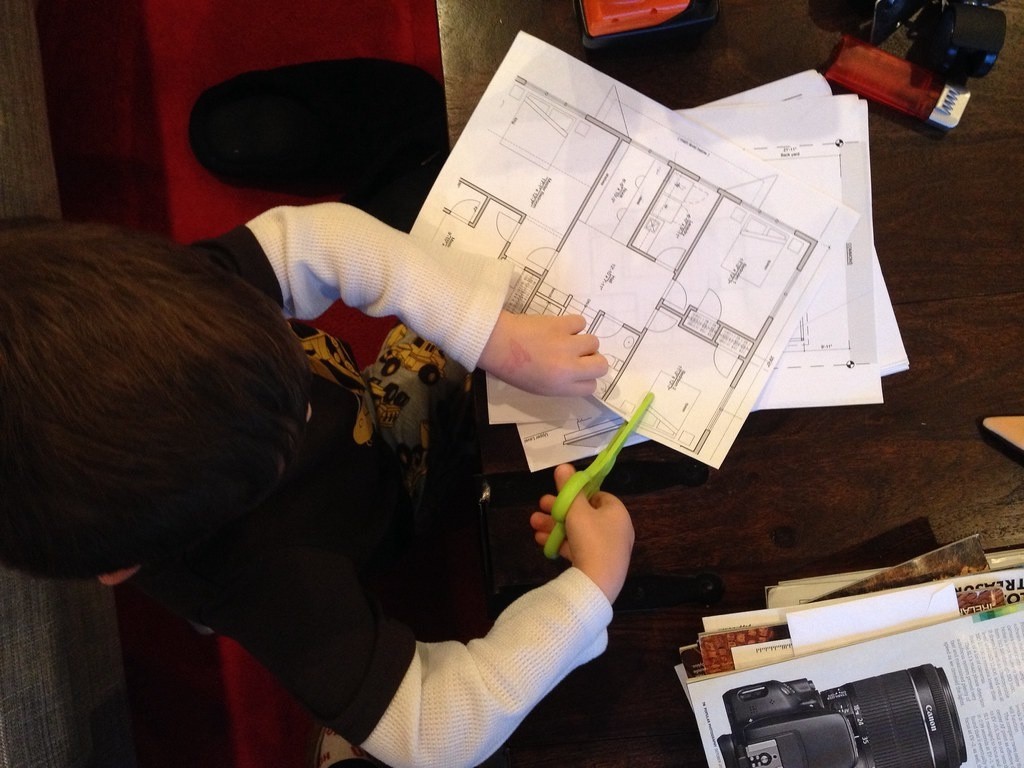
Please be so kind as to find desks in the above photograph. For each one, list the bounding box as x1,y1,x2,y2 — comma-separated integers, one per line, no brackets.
431,0,1023,768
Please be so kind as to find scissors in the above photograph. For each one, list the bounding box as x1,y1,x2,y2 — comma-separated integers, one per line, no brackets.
544,390,655,560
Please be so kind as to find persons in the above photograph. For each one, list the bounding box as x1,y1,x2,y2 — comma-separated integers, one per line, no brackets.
0,201,635,768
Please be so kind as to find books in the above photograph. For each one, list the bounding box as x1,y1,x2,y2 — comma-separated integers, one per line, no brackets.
674,535,1024,767
